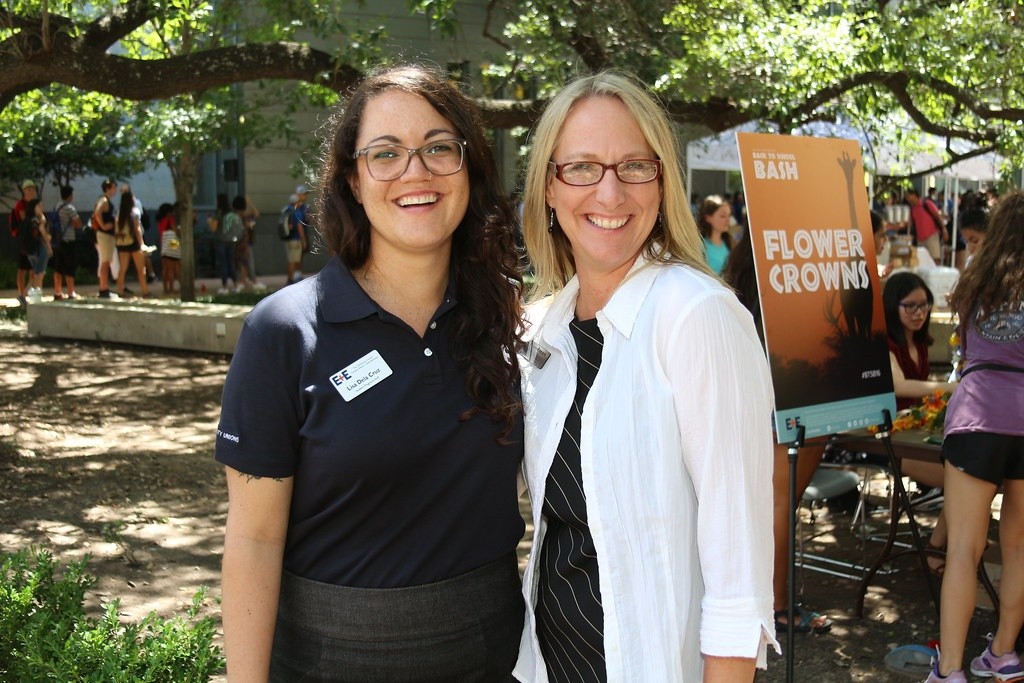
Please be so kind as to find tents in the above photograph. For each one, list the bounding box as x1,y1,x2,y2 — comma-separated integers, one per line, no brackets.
686,118,1013,209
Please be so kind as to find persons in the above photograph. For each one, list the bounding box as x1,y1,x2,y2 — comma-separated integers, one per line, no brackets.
89,180,180,297
512,72,786,683
11,181,83,305
212,60,525,683
885,272,956,575
277,184,310,285
695,185,834,632
924,195,1024,683
865,183,994,270
209,194,267,295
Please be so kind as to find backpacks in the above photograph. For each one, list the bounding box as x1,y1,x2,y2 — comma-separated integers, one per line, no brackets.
8,208,22,239
278,207,295,241
45,203,72,250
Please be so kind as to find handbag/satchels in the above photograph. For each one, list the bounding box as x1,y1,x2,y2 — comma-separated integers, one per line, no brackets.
161,229,181,260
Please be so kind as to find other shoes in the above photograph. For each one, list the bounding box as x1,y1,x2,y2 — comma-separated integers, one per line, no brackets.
928,556,946,576
98,274,268,301
17,295,28,305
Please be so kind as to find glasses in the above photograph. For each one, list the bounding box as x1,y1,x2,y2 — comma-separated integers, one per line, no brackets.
548,158,662,187
899,302,929,315
351,139,469,182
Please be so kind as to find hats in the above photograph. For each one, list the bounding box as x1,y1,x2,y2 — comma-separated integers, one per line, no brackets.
296,185,310,194
22,179,37,189
289,194,301,203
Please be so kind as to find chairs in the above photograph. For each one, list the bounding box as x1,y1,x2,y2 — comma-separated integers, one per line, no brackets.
799,456,941,579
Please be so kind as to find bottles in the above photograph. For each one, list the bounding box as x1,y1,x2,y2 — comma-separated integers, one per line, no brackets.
887,205,909,223
27,287,42,304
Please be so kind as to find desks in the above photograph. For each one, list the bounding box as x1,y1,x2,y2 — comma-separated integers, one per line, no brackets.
835,429,1001,620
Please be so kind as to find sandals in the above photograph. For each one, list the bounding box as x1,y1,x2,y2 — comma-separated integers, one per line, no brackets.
774,605,834,634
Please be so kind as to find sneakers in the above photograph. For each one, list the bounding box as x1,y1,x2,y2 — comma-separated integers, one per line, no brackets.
923,644,969,683
969,632,1024,681
